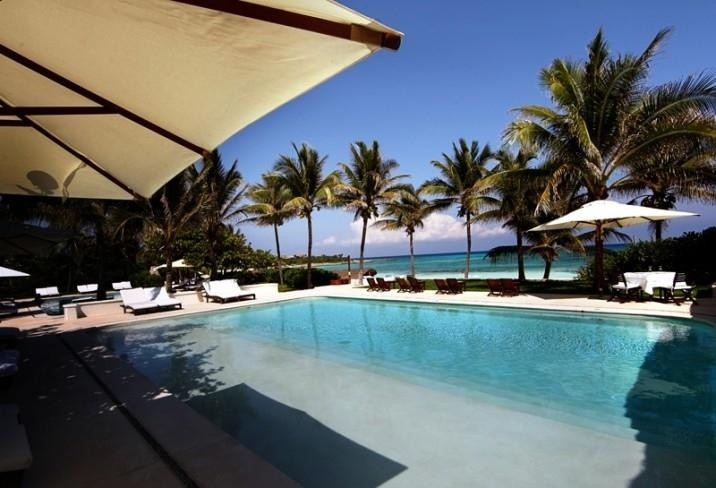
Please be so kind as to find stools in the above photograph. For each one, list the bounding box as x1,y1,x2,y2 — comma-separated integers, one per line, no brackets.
0,326,34,487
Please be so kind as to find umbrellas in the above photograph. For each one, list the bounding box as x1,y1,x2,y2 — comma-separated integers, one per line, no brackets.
527,200,701,294
155,259,194,284
0,0,403,200
0,266,30,276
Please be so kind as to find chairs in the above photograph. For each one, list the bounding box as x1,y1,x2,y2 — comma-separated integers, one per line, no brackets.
76,282,98,294
35,284,62,298
112,279,132,290
118,286,182,317
605,269,700,306
366,277,390,292
487,277,520,298
202,278,256,305
395,275,426,293
434,277,465,295
